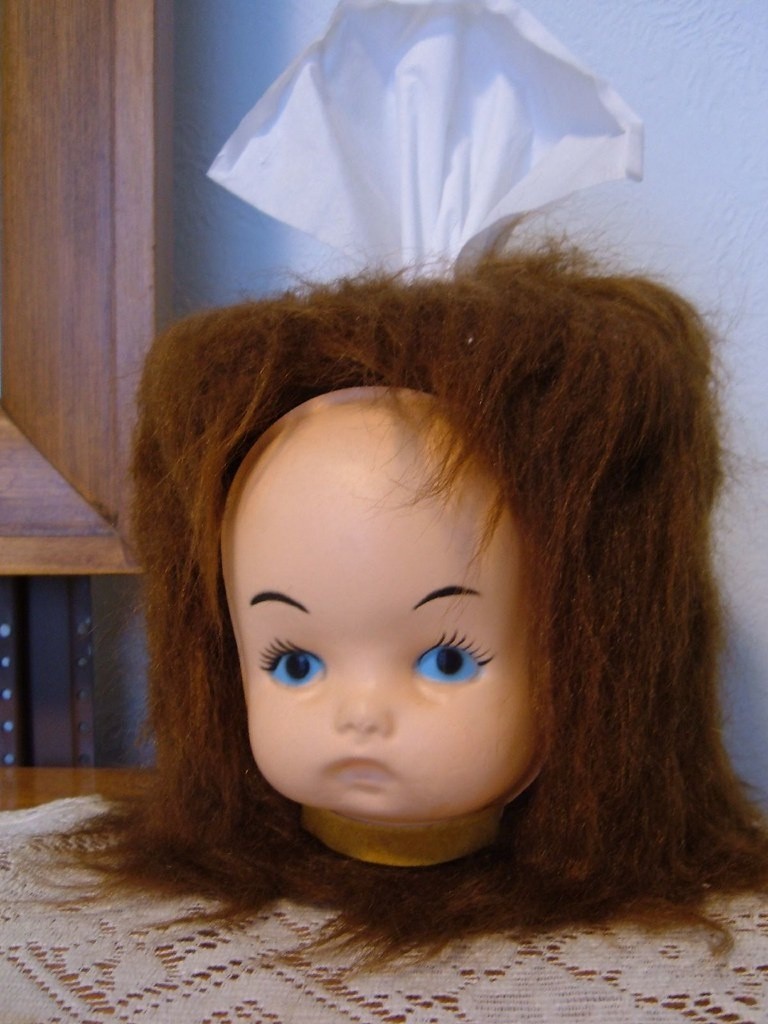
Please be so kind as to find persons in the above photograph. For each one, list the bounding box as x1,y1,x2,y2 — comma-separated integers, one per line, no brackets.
70,264,753,949
